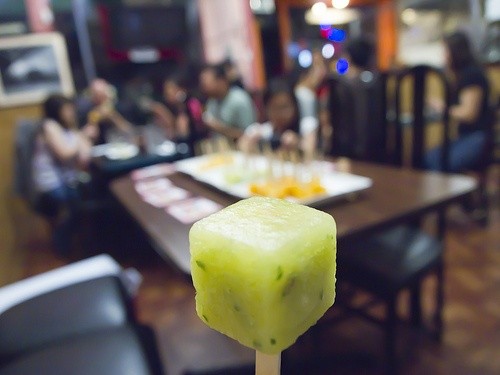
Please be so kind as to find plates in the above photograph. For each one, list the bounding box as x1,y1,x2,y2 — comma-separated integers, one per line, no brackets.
176,150,371,206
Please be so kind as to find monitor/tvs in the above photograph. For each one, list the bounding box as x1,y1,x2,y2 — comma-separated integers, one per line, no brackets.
0,32,75,107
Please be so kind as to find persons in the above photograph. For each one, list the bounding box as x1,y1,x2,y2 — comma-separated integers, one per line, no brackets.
26,66,256,261
422,30,495,225
237,78,319,159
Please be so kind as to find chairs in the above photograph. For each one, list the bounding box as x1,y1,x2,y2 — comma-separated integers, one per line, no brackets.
8,118,69,253
462,63,500,230
297,63,453,375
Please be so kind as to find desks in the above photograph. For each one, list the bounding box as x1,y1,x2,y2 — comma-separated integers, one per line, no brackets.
107,152,479,356
88,150,195,255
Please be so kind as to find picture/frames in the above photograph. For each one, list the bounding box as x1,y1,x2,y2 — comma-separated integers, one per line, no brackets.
0,30,76,111
